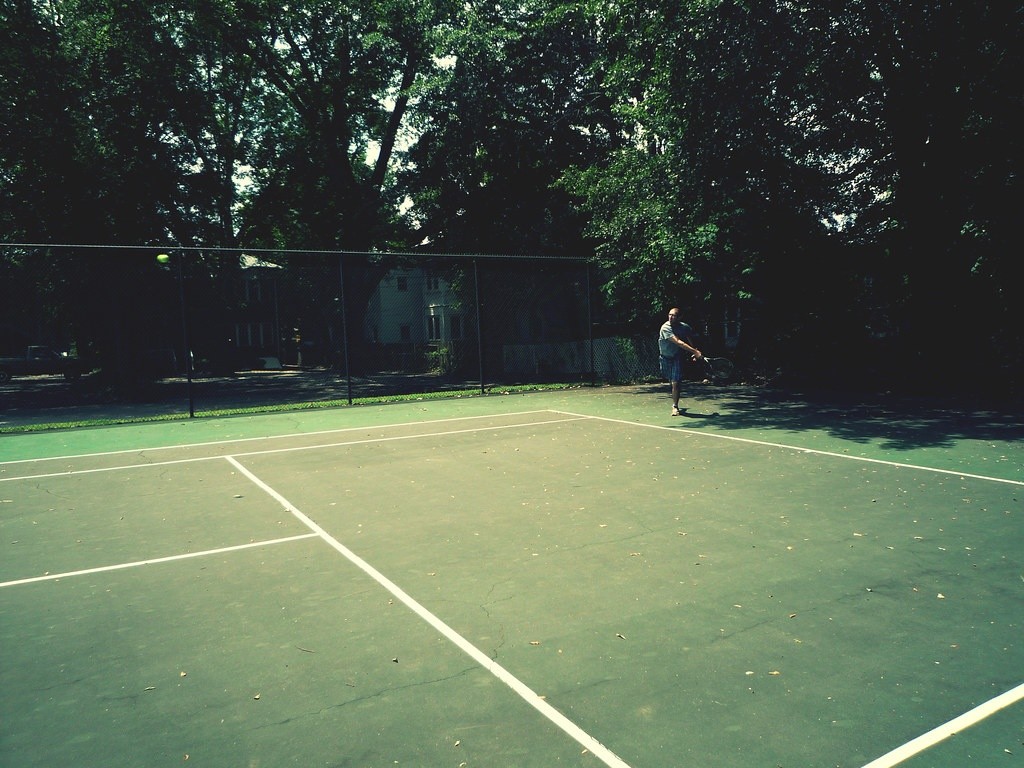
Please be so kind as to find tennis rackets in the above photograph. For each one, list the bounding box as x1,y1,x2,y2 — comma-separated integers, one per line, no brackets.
702,355,733,379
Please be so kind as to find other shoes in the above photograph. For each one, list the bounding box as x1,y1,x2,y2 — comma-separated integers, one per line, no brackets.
671,406,679,416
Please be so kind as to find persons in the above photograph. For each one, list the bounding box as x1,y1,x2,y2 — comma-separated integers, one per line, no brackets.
657,308,703,416
